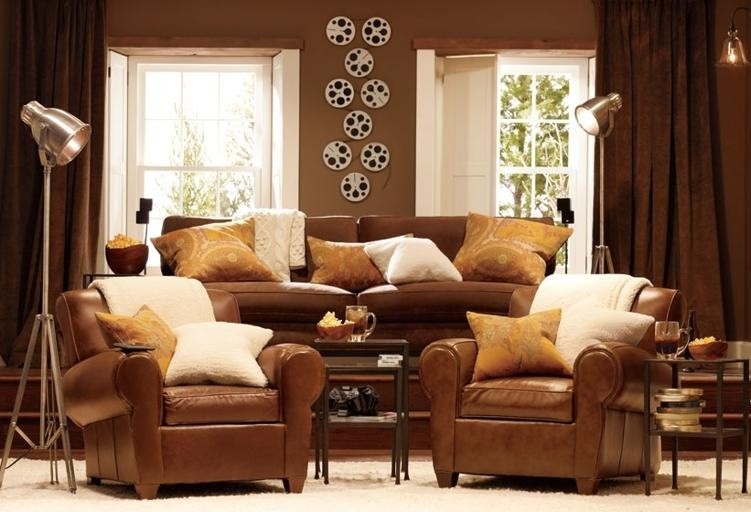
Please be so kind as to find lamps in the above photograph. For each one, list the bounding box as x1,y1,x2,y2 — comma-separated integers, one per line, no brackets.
0,101,92,493
715,7,751,68
575,93,623,273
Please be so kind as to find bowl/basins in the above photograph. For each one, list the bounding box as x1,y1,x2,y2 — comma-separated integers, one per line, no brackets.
104,245,150,275
688,340,729,361
315,324,355,344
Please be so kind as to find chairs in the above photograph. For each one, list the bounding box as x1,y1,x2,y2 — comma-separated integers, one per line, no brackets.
55,287,327,500
419,273,686,494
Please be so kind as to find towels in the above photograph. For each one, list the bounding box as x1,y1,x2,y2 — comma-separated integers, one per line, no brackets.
232,208,307,282
88,276,216,332
530,274,654,313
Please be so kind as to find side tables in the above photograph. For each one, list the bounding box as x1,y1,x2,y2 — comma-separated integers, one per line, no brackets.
313,335,410,485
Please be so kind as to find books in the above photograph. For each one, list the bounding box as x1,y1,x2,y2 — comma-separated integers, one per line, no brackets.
652,387,707,433
329,410,404,423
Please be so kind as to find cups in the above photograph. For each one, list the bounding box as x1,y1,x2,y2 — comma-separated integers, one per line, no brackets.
346,305,377,343
655,321,690,359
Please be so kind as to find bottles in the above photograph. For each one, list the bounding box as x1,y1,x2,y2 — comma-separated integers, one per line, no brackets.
685,311,699,359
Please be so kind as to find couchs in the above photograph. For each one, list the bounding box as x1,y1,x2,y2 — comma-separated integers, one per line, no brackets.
163,216,556,349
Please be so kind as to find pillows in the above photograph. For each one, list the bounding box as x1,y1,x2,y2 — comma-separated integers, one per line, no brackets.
555,306,655,376
95,304,176,384
453,212,574,285
307,233,412,289
165,322,273,388
363,238,462,283
150,217,280,282
465,308,575,382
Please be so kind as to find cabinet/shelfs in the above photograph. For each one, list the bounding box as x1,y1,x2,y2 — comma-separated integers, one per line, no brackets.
645,356,750,499
